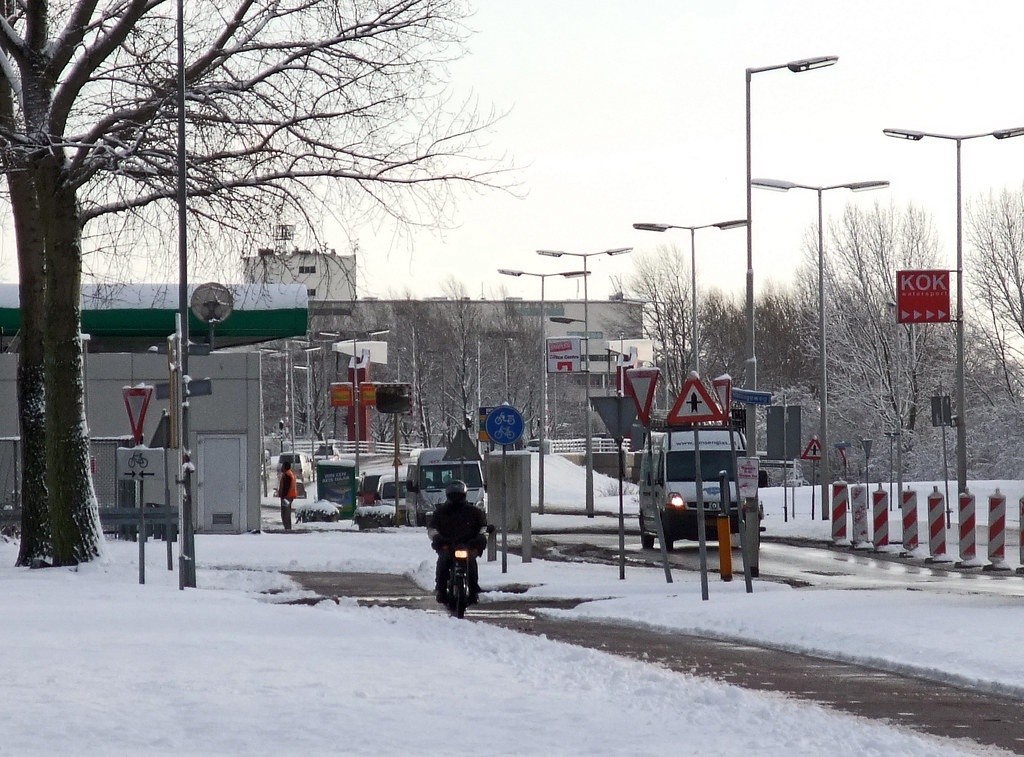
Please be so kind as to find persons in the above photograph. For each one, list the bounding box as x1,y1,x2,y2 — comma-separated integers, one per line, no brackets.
428,480,488,604
279,462,298,530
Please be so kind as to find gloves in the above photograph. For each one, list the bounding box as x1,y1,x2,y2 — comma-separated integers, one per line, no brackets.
431,534,446,551
282,499,290,506
475,534,488,550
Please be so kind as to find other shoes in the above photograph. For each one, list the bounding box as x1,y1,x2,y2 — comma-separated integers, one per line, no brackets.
468,593,479,606
436,589,448,604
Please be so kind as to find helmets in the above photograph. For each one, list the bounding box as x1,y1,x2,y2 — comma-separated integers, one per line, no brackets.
445,480,468,500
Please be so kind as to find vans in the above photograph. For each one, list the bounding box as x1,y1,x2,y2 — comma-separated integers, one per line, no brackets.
378,475,405,523
640,431,763,552
277,452,314,483
406,446,488,527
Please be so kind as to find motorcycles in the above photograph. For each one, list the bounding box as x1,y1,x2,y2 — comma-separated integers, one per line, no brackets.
423,523,496,619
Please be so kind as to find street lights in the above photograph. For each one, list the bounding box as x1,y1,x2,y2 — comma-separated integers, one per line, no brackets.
497,266,592,514
633,219,749,370
744,54,839,570
536,248,632,518
750,179,891,521
883,127,1024,489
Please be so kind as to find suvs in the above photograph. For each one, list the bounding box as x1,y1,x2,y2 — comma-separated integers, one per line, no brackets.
311,445,341,471
358,475,380,511
259,329,390,477
